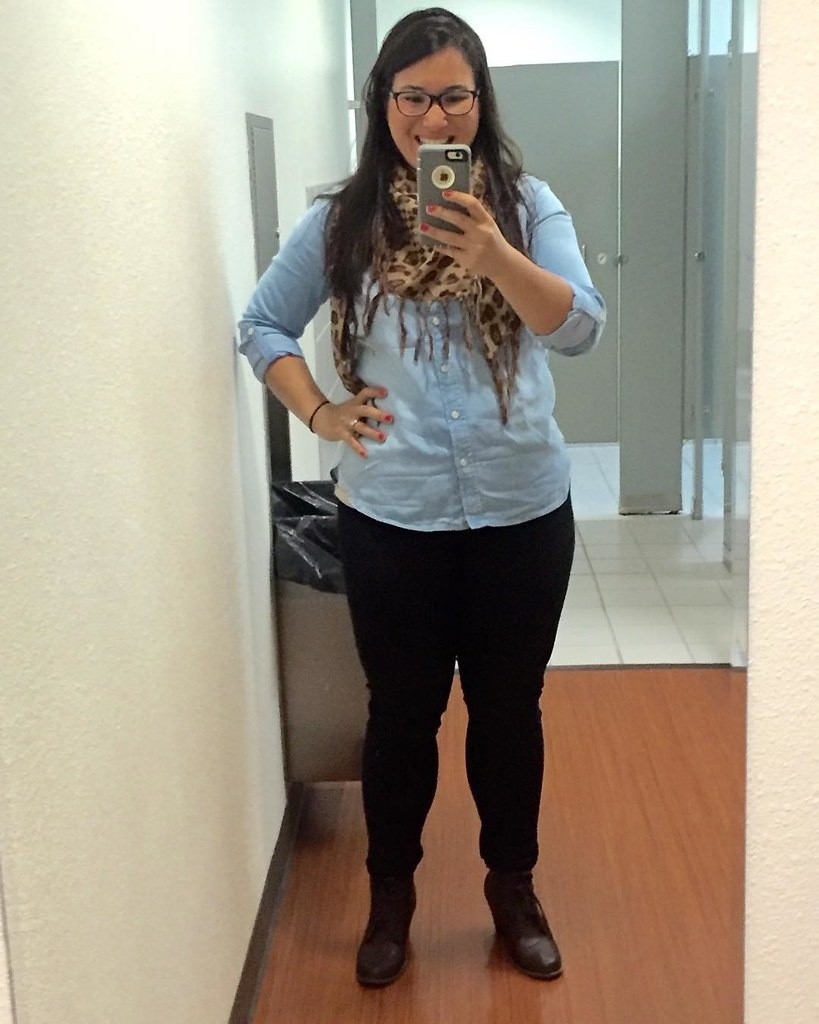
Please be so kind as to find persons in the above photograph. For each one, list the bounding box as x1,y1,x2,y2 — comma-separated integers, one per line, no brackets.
238,6,609,990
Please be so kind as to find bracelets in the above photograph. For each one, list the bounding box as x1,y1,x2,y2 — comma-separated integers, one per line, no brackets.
308,400,330,433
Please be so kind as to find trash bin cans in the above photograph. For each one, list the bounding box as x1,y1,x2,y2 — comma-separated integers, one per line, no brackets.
271,480,370,783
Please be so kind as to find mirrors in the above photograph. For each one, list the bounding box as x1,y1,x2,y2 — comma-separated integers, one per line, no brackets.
0,0,759,1024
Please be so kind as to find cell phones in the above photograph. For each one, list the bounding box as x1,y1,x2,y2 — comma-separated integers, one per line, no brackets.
416,143,472,245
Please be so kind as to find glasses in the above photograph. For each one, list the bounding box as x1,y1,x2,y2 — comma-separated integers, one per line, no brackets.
388,87,481,117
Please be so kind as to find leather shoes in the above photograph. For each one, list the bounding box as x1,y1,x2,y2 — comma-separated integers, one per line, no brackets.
356,874,416,987
484,869,563,980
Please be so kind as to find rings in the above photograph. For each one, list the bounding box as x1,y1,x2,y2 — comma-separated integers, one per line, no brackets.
350,420,360,431
